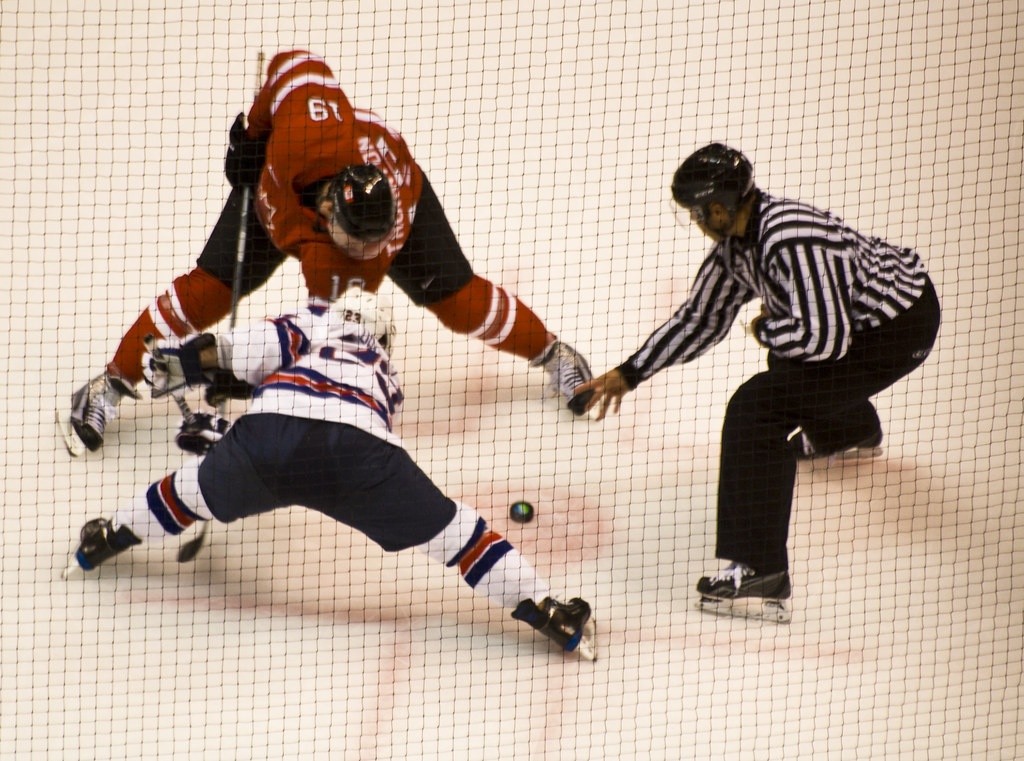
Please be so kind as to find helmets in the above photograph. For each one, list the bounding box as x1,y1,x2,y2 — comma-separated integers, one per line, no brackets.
670,142,757,213
331,164,397,259
318,285,391,355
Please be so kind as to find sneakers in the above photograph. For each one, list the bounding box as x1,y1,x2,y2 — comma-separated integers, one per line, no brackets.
696,559,793,624
528,338,601,420
786,419,885,461
509,597,595,662
64,517,142,577
56,371,145,456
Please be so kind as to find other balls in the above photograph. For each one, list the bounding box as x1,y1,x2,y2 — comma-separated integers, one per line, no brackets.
509,500,536,522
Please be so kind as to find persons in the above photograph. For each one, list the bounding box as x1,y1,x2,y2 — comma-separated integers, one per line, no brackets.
55,53,591,458
63,284,599,659
576,140,941,626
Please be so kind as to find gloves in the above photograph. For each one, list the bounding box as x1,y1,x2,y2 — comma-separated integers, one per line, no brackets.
224,114,265,189
139,333,218,401
175,414,232,457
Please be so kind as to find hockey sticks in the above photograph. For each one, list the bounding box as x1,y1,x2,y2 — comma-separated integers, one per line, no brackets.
143,333,199,428
177,186,253,563
